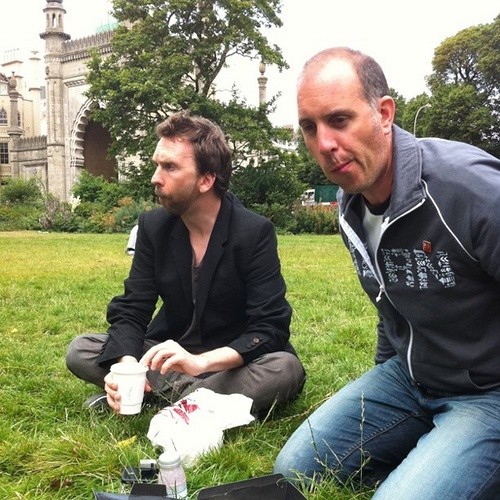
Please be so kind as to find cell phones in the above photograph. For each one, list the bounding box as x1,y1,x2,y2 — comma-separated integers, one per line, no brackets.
122,467,160,484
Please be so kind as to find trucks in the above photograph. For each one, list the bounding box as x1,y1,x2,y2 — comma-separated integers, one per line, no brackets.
296,184,342,207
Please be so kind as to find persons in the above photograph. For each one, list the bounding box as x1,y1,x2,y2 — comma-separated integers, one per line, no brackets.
272,44,499,500
65,107,307,417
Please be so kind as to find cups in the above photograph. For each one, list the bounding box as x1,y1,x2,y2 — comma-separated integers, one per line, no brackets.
109,362,149,415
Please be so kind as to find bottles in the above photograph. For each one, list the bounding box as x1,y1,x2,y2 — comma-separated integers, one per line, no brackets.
158,452,187,499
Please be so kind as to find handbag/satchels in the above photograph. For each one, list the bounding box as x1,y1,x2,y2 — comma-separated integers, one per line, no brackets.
143,388,255,465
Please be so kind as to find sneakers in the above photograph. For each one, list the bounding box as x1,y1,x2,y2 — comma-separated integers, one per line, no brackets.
84,392,153,413
